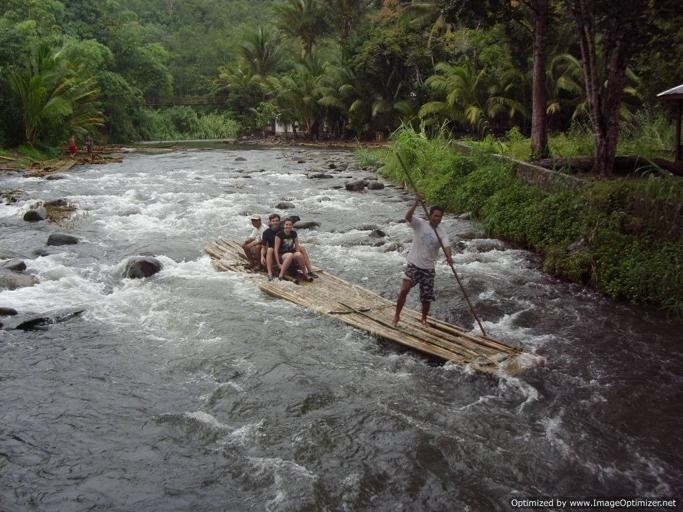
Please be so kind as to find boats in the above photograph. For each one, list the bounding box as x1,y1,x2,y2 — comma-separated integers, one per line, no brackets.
203,237,545,381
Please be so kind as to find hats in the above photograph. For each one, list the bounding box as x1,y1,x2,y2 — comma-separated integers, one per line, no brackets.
248,214,261,221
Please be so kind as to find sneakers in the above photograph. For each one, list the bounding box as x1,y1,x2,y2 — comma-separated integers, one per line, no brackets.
244,263,319,284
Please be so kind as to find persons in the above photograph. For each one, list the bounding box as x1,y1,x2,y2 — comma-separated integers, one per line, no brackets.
273,218,314,282
390,191,455,329
239,213,269,272
259,213,319,282
